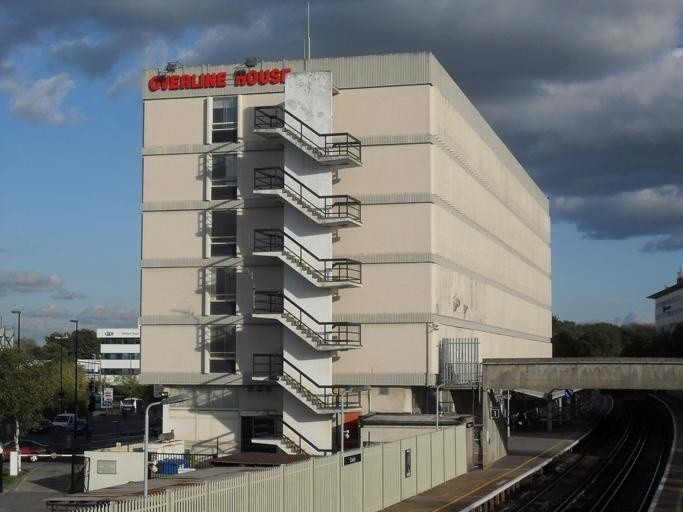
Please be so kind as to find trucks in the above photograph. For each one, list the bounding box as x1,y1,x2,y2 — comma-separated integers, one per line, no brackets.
52,413,87,435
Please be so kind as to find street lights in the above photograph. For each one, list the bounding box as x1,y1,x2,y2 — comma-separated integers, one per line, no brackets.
10,310,20,352
54,336,69,413
143,392,191,499
435,380,460,430
340,385,372,454
68,319,78,449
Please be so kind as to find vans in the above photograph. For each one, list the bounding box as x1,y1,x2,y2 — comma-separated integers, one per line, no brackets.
119,397,144,418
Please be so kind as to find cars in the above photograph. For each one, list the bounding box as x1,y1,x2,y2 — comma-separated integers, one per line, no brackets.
0,439,49,463
29,419,51,435
